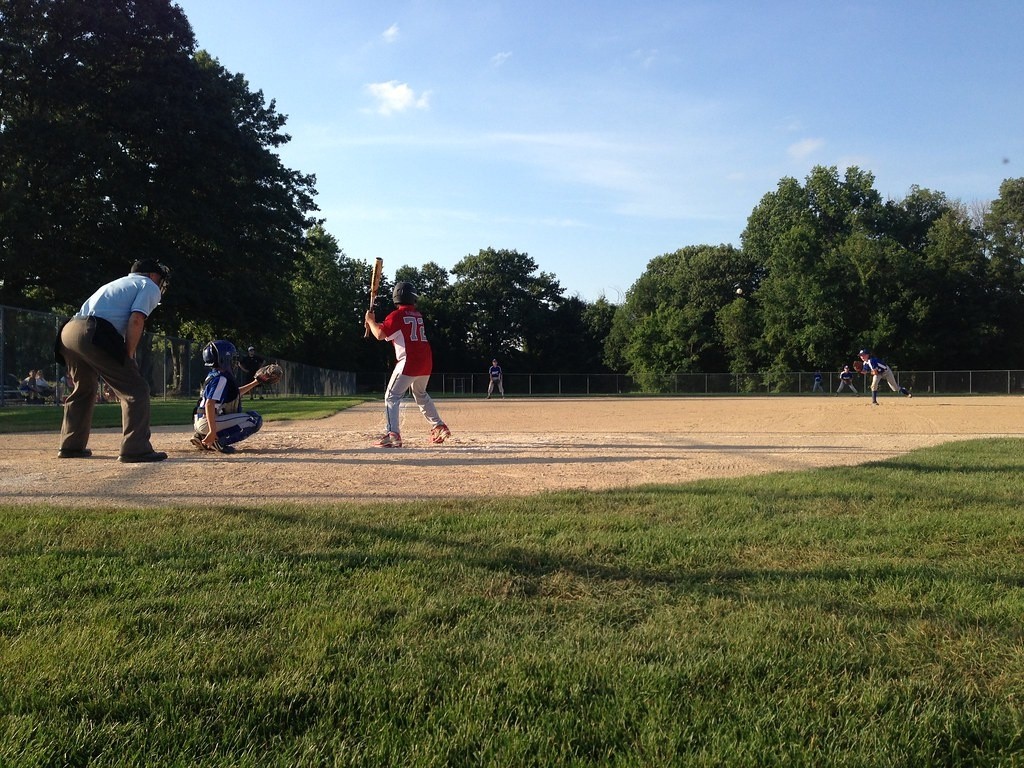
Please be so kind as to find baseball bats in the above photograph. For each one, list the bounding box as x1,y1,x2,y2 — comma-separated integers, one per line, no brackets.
364,257,384,339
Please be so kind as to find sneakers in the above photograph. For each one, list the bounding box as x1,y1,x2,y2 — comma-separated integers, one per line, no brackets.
210,440,233,453
190,432,209,450
428,424,450,444
371,432,402,448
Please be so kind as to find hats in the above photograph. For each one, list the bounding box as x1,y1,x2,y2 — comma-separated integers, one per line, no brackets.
844,366,849,369
248,347,254,351
857,349,869,356
492,359,497,362
131,259,161,273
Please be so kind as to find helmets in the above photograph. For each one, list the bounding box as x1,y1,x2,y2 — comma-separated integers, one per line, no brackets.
393,282,418,304
203,340,238,371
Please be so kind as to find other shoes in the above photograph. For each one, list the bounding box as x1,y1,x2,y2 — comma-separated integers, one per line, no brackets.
872,402,879,406
118,452,167,462
58,449,92,458
906,392,912,398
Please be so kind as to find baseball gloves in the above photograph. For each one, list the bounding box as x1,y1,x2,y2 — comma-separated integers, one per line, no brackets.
254,363,284,387
493,379,501,384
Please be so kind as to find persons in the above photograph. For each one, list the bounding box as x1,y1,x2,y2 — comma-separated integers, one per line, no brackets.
812,370,824,392
854,349,912,406
364,283,451,448
238,346,265,400
835,366,859,397
190,340,282,453
19,369,121,404
58,259,170,464
485,359,505,399
